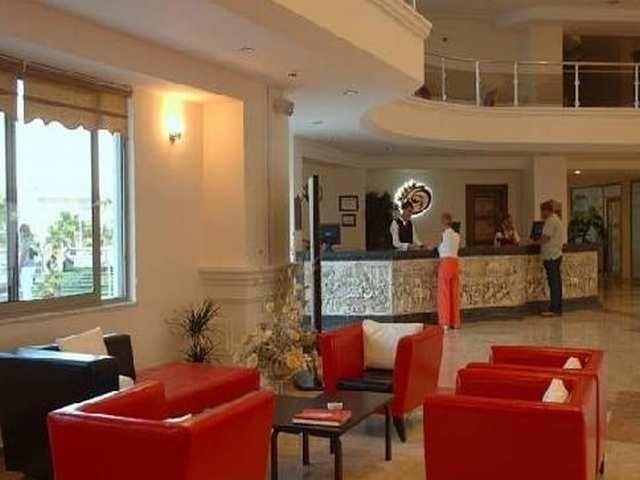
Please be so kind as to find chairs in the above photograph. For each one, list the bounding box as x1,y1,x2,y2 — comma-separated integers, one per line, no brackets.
47,380,277,479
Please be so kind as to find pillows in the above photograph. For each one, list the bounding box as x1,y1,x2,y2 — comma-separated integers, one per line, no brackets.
362,318,424,370
119,374,134,389
55,326,109,355
562,356,582,369
543,378,569,403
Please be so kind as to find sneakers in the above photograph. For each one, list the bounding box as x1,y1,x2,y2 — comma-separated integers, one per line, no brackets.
540,310,561,317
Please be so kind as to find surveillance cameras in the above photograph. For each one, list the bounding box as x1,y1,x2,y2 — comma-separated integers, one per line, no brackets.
274,98,294,117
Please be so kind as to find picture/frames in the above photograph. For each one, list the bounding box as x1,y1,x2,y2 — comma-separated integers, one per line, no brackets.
342,214,356,227
339,195,359,211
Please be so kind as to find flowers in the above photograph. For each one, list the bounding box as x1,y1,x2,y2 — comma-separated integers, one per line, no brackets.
232,299,311,375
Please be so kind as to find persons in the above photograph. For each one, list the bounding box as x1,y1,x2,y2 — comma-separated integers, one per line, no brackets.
494,213,528,250
389,201,424,250
441,214,463,248
526,201,564,317
427,221,460,330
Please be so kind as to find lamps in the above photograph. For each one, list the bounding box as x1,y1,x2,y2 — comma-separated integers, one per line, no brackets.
163,109,184,145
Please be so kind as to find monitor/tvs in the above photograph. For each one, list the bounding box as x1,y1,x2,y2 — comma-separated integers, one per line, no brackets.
532,221,544,239
318,223,341,252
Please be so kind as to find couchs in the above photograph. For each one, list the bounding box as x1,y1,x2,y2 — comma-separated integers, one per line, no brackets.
465,343,610,476
423,368,599,479
320,321,444,442
2,333,260,479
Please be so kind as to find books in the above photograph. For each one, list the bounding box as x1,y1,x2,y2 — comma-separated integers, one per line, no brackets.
293,409,351,425
